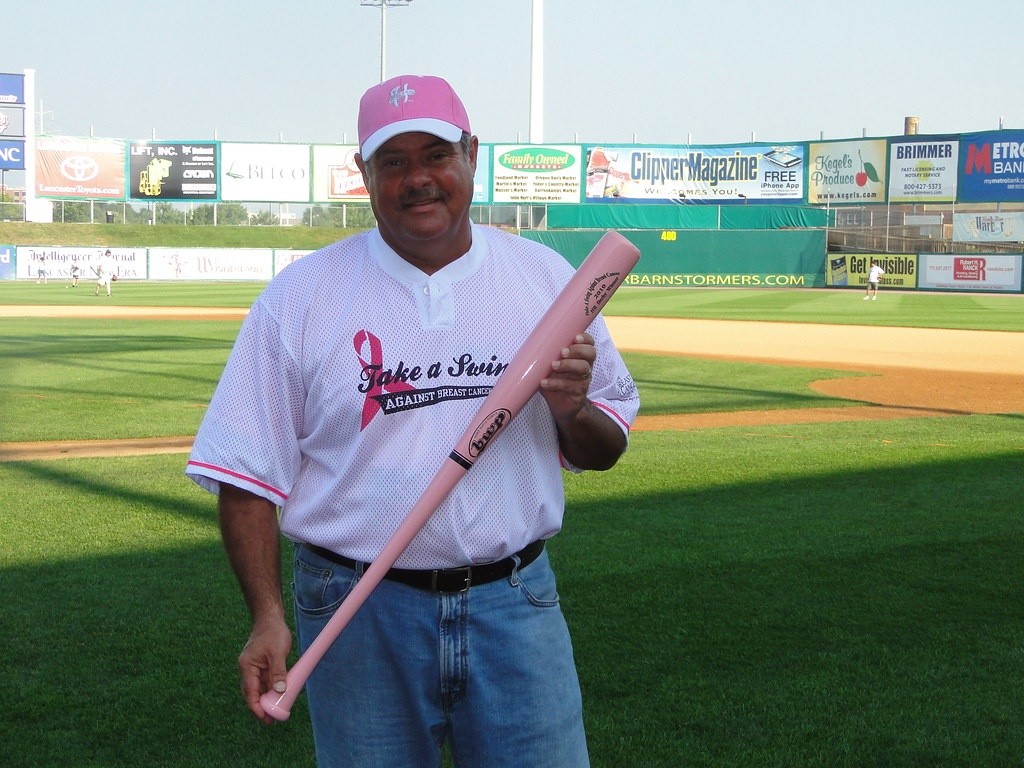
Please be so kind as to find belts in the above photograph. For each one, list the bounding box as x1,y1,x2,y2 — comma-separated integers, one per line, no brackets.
306,539,546,596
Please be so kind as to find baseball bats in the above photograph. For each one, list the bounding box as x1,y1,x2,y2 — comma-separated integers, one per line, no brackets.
259,228,642,722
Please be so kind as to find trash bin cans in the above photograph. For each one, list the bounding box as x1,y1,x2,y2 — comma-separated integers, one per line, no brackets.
106,210,115,223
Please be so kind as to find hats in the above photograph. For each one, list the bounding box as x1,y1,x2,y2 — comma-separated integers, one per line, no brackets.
359,75,472,161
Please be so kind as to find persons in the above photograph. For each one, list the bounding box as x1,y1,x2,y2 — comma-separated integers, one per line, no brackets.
863,262,884,300
185,75,641,768
70,259,80,287
37,257,47,284
96,250,116,297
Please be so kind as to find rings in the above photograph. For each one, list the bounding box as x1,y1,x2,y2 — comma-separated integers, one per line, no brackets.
582,369,591,377
